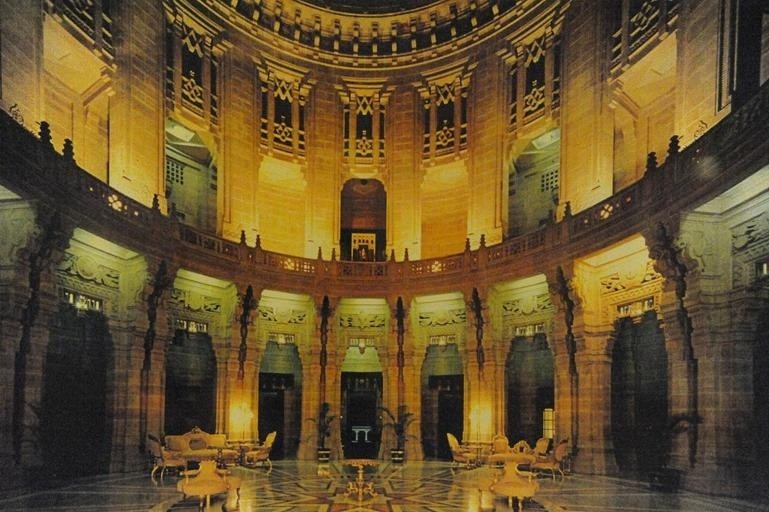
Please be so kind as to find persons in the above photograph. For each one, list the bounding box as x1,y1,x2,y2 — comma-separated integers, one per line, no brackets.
664,416,696,495
